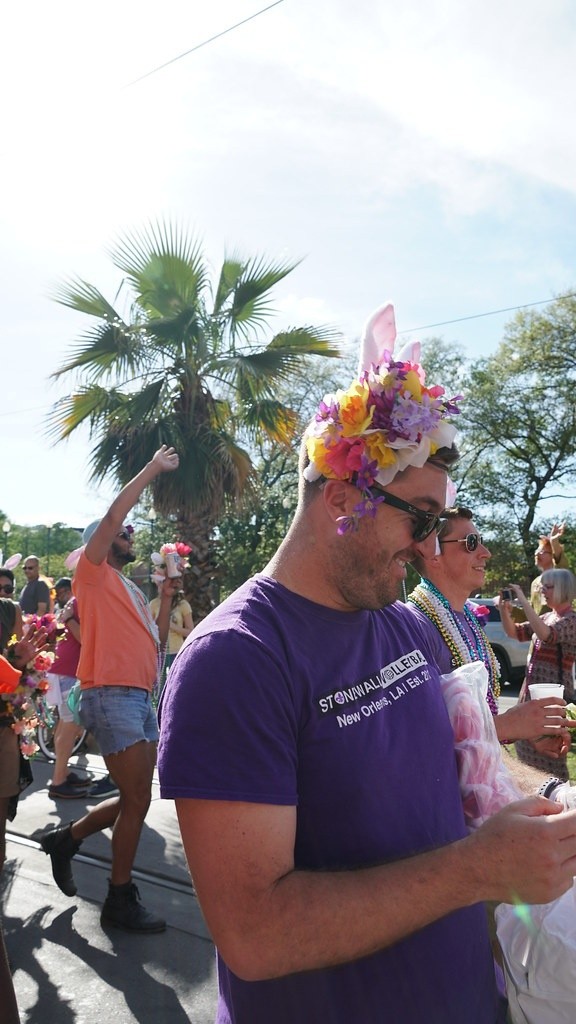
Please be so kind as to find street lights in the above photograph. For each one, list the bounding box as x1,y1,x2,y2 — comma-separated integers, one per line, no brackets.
2,521,10,555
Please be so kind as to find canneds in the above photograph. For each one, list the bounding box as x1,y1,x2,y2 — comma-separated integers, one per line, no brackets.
166,552,182,578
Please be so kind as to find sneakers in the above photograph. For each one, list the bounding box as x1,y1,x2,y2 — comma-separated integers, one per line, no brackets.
87,777,121,798
65,772,92,787
48,782,87,800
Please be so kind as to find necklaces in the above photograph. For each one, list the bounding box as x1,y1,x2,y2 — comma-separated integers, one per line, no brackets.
407,578,510,745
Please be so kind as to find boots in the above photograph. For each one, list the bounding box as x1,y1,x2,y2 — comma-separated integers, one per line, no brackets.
101,877,167,934
40,819,84,897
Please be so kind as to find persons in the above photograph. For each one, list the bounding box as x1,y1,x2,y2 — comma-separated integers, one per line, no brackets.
492,519,576,782
0,444,192,930
406,507,576,759
148,542,193,697
158,303,576,1024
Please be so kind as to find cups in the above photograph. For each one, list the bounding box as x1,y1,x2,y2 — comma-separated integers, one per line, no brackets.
528,683,565,728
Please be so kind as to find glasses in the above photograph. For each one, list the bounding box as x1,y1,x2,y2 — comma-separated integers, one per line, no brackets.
439,533,483,552
541,586,553,591
116,531,131,539
0,584,14,594
535,549,551,555
319,476,448,542
23,566,37,570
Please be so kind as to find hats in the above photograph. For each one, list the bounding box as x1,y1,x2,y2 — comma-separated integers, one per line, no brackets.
82,519,103,544
47,577,73,589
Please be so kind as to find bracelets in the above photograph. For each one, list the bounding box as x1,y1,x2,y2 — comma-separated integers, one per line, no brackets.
538,776,563,798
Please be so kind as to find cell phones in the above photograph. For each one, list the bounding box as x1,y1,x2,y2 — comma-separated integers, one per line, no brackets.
503,589,513,601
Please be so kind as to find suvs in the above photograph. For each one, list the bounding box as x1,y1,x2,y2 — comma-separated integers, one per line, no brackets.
465,598,533,694
